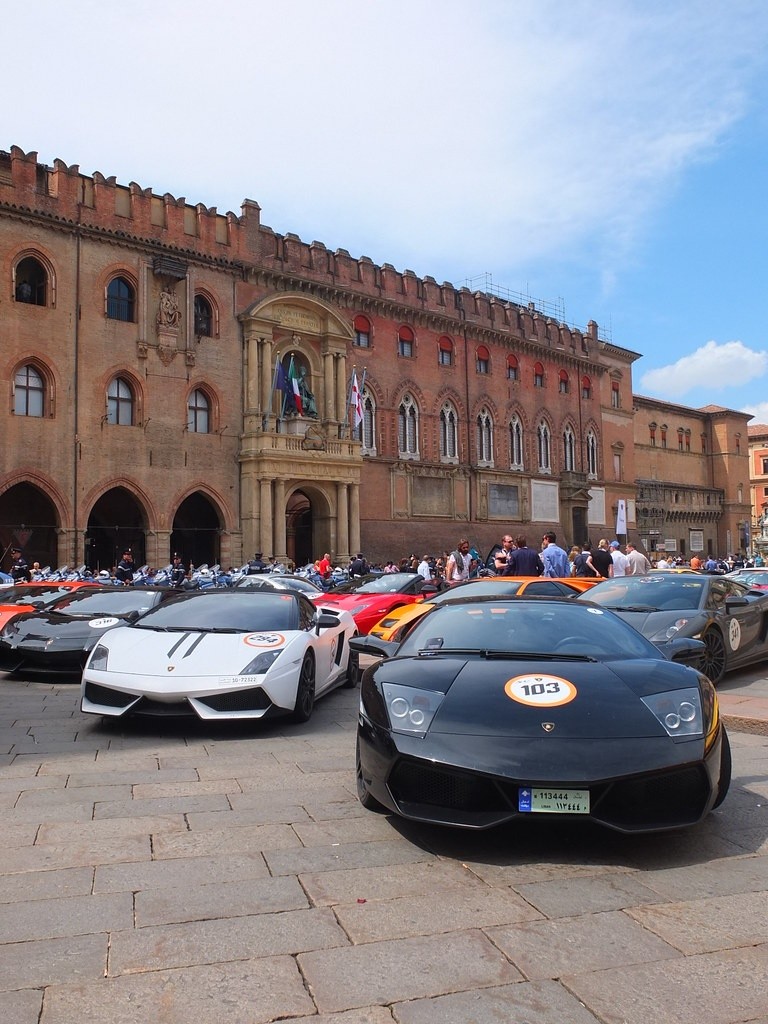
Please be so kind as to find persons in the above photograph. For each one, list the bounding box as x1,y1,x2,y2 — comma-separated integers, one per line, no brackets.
8,531,767,591
17,280,31,303
160,287,180,326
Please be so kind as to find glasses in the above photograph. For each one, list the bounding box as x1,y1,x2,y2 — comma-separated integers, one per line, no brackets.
463,546,468,549
506,540,513,543
542,537,547,540
172,557,181,561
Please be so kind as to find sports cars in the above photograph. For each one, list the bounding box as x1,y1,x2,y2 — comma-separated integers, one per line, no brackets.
0,566,768,644
79,586,358,725
0,586,184,679
574,572,768,686
347,594,732,835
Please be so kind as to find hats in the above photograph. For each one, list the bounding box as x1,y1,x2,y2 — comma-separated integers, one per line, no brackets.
254,552,263,556
357,553,364,556
268,556,275,560
11,545,23,553
609,541,620,549
121,547,134,555
350,557,356,560
173,552,182,558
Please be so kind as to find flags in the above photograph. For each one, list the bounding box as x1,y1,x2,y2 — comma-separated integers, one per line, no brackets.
290,360,302,414
275,362,294,406
350,372,366,427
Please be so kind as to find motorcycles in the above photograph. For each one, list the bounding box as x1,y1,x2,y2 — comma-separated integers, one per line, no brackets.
0,561,350,592
